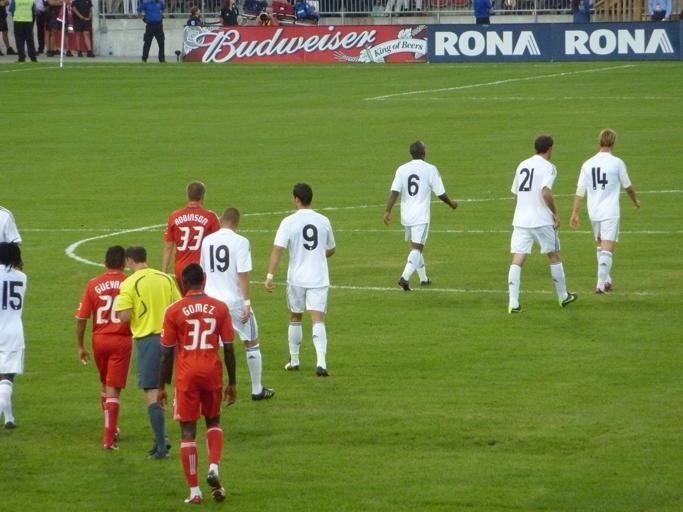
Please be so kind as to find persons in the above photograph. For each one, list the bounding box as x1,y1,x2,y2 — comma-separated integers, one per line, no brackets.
138,2,165,63
42,1,66,57
505,136,577,312
0,1,15,56
198,208,275,401
1,242,28,427
116,248,186,458
647,0,671,21
385,139,457,290
185,5,219,27
10,0,37,61
165,181,221,292
157,266,237,503
76,246,130,449
0,206,21,244
268,184,336,376
570,130,639,292
571,0,589,22
70,0,94,57
222,0,237,24
257,8,279,25
473,0,491,23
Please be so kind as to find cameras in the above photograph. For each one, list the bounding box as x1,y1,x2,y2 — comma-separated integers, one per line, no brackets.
259,13,267,23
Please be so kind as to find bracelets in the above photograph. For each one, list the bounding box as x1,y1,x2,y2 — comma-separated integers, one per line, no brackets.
241,300,251,307
266,274,272,279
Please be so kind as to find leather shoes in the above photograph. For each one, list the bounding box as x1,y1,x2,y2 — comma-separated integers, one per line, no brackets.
38,50,44,53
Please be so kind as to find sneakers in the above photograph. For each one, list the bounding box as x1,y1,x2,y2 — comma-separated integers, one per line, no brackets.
206,472,225,502
5,419,16,428
421,279,432,285
144,452,169,460
507,304,521,314
398,277,410,291
7,47,18,55
605,282,612,291
31,57,37,61
104,441,120,450
184,494,201,505
252,387,275,400
0,51,4,55
284,363,301,371
54,50,61,55
47,51,54,57
66,50,73,56
559,291,578,307
18,58,25,62
78,51,83,57
115,427,122,441
87,51,95,57
316,367,329,376
148,438,172,455
595,287,606,294
